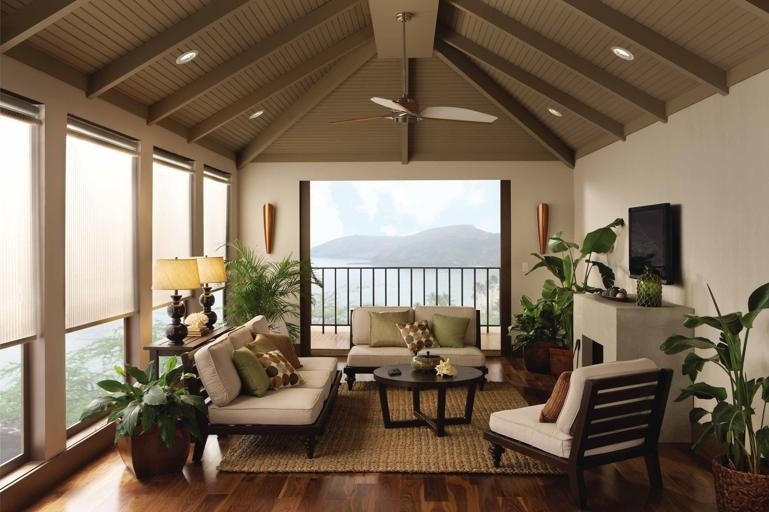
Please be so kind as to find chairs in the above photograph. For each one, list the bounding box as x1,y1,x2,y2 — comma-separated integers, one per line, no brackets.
484,357,674,511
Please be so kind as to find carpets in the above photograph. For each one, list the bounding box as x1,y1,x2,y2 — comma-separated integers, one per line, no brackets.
217,384,574,474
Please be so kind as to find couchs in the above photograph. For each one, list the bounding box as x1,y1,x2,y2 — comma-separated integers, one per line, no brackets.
180,316,343,463
343,305,490,392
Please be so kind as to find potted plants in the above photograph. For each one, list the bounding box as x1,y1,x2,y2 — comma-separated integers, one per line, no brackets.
659,284,768,512
525,219,622,375
507,292,553,374
78,354,208,482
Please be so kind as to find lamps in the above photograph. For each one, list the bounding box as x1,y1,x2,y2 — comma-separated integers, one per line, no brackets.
263,203,275,253
193,257,226,332
536,203,550,254
149,258,198,345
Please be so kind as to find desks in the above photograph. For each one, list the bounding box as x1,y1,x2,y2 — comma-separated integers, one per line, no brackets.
142,326,237,439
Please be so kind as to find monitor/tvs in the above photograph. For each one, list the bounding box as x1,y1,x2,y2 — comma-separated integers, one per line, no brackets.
629,203,675,285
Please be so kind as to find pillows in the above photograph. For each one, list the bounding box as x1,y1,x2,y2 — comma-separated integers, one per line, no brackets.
370,311,411,347
246,335,274,360
429,311,469,347
266,335,302,370
259,350,304,390
540,370,572,422
395,319,441,351
230,347,270,397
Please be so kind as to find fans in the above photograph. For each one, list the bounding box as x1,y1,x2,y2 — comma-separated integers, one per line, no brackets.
327,10,497,126
